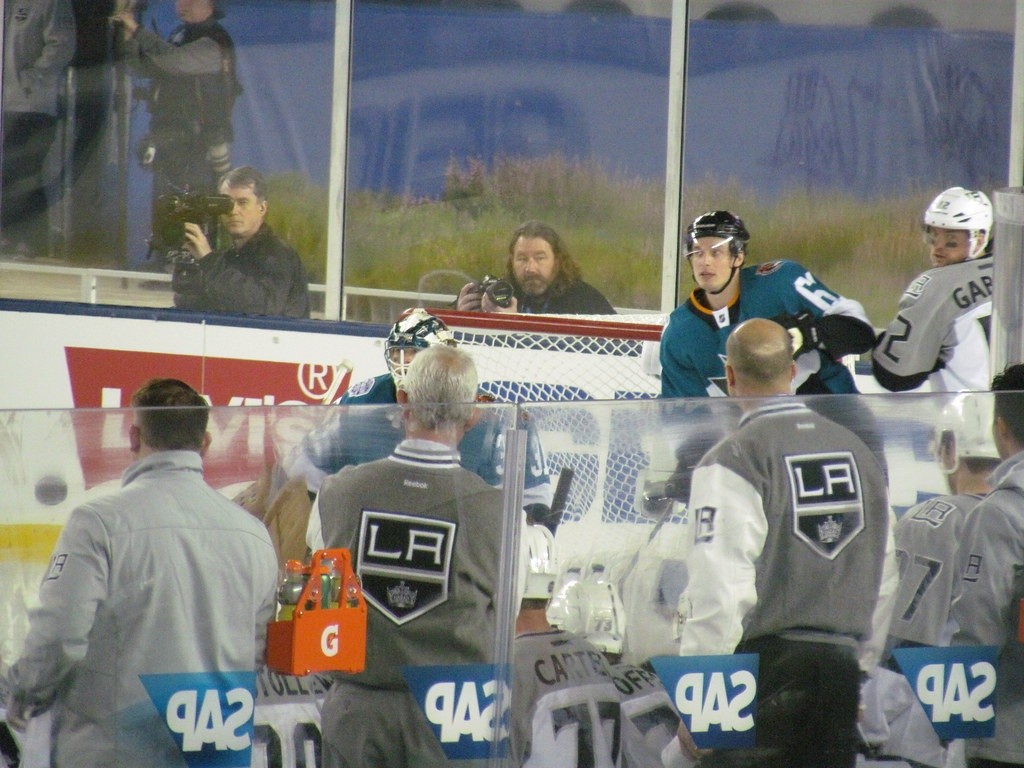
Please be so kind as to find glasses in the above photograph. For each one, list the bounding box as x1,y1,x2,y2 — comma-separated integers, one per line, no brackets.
925,234,968,249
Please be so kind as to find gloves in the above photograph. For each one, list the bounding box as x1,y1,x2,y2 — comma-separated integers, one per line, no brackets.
666,433,718,503
661,735,699,768
773,309,822,360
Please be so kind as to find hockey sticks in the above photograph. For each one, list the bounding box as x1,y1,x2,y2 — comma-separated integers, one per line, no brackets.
616,494,678,595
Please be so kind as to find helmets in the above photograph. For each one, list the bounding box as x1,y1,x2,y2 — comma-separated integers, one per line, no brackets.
384,308,457,390
544,579,627,655
520,524,555,600
924,187,993,259
687,211,750,260
934,390,1001,460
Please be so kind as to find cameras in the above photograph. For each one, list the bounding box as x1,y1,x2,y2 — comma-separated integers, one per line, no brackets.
468,275,514,310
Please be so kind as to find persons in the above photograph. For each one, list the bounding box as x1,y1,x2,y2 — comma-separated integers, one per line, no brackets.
871,186,996,394
4,378,278,768
172,164,310,320
442,219,617,315
231,308,1024,768
115,0,244,272
0,0,76,257
659,209,888,501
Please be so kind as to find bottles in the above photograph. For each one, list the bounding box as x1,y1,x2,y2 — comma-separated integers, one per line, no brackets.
270,556,360,622
81,269,96,304
